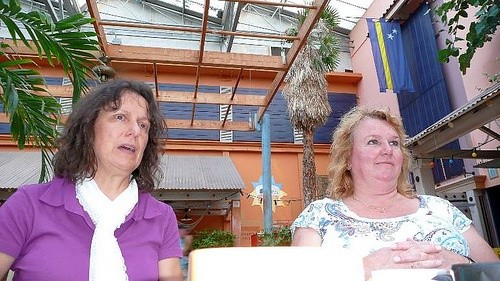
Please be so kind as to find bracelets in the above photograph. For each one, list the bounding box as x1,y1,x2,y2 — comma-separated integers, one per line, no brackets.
463,255,477,264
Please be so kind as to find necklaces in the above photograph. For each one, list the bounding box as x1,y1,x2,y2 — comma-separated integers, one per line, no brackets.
351,192,399,214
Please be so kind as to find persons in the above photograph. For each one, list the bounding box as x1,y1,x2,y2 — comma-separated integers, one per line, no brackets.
290,106,500,281
0,78,183,281
177,225,193,281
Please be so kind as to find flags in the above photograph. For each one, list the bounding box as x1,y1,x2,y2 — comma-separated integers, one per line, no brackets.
366,18,416,94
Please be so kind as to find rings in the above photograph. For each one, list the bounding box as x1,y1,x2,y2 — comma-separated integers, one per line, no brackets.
411,261,414,268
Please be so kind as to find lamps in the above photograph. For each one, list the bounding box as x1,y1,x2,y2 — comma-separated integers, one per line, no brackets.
181,208,193,221
